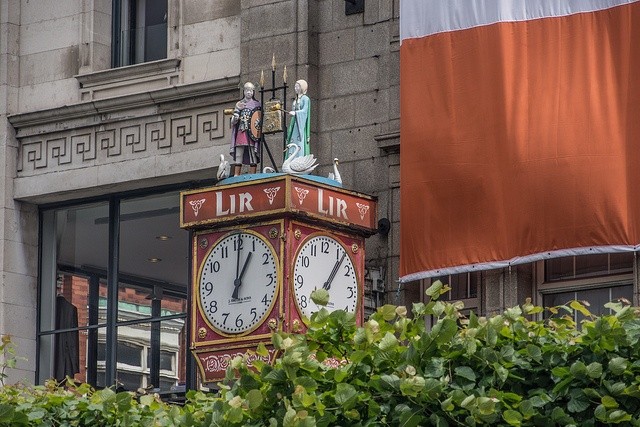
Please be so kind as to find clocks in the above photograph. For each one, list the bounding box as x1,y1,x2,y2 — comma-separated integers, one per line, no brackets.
291,230,361,331
196,227,280,338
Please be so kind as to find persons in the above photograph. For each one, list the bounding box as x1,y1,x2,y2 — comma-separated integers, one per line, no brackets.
231,80,263,176
287,79,311,157
